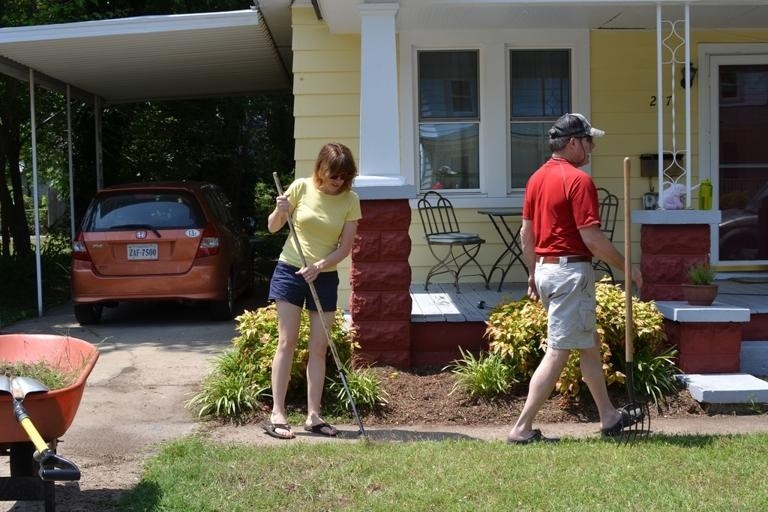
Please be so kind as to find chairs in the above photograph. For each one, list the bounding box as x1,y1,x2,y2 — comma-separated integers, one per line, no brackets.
419,191,488,293
591,188,619,284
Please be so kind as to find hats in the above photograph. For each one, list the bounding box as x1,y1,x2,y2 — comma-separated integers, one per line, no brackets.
548,112,606,138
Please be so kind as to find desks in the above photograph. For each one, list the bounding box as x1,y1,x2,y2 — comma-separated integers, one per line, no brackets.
477,206,529,293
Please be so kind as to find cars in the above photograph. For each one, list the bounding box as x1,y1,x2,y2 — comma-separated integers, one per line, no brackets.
719,181,768,259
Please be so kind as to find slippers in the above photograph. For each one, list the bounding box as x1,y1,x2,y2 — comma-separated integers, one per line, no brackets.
261,418,295,438
600,408,645,436
506,428,560,443
304,422,338,436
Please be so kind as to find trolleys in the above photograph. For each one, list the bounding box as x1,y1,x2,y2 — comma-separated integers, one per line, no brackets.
0,333,99,512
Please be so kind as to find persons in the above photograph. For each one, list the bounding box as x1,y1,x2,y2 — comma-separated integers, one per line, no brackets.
507,112,645,446
261,142,362,439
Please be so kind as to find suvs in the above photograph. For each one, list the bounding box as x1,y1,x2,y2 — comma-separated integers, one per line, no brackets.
71,183,255,328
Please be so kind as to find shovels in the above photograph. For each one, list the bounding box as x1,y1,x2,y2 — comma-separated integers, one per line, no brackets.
1,377,81,483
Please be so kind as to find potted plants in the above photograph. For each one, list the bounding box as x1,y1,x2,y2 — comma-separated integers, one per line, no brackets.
682,264,719,306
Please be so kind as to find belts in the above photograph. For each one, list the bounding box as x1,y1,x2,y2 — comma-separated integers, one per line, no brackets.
534,255,590,264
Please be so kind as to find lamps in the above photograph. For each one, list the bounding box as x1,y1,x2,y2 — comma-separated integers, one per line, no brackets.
681,61,697,87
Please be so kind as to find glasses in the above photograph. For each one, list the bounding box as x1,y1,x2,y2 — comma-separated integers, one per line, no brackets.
328,174,347,180
